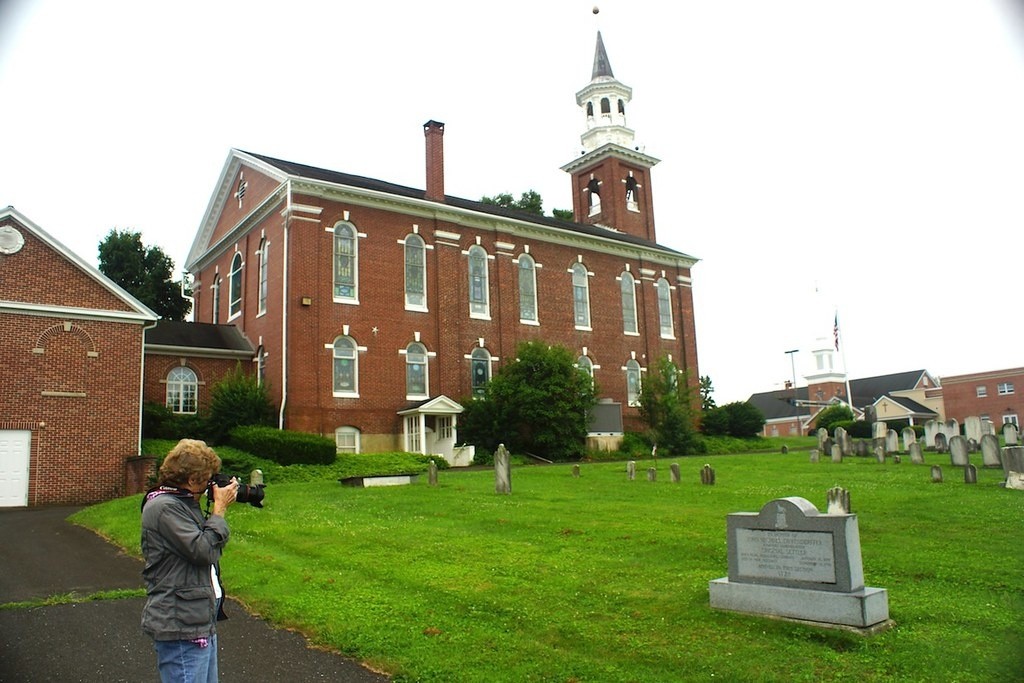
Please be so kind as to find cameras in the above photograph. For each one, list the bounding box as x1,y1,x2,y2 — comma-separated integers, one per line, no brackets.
208,473,267,508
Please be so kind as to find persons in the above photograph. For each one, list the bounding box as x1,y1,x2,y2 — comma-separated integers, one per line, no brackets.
140,438,238,683
